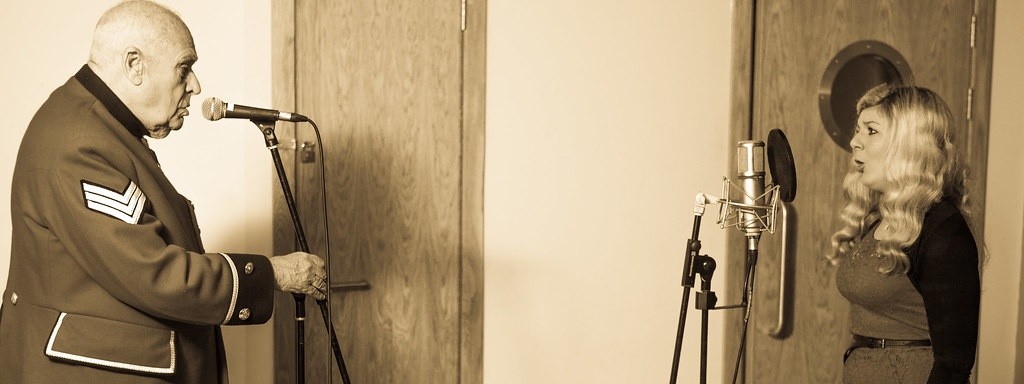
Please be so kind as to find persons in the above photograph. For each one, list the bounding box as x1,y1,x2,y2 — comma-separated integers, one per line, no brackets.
0,0,331,383
825,82,986,384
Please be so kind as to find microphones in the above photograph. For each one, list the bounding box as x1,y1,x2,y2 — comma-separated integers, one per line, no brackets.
201,97,309,123
738,139,768,267
